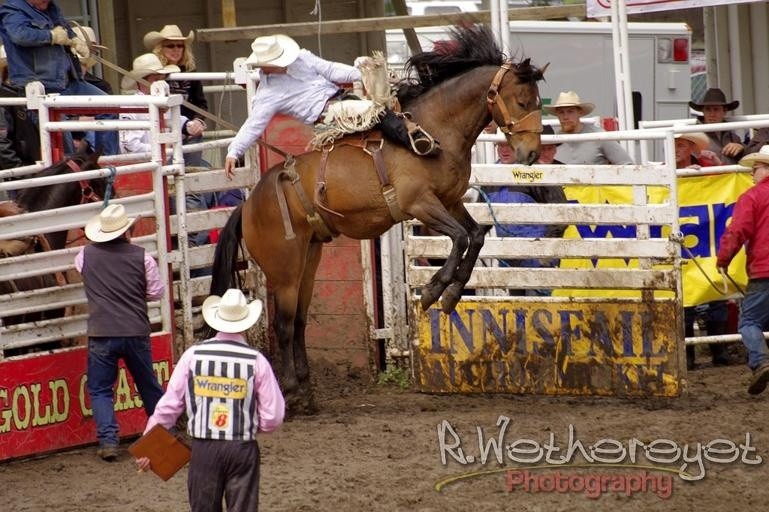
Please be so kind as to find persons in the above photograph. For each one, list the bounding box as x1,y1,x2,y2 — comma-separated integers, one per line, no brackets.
135,289,285,512
475,88,769,395
0,1,208,248
225,33,443,181
73,204,178,459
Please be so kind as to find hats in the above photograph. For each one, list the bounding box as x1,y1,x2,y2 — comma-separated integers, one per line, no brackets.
202,288,263,333
688,88,739,111
143,24,194,51
121,53,181,90
739,144,769,166
72,26,110,50
245,34,300,66
84,203,135,242
673,123,710,150
543,90,596,117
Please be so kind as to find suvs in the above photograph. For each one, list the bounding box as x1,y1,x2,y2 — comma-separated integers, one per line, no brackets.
406,1,477,17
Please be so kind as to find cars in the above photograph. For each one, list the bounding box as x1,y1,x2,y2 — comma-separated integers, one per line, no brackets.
506,0,566,20
691,47,707,102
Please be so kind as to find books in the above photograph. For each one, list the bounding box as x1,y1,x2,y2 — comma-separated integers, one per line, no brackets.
127,423,191,481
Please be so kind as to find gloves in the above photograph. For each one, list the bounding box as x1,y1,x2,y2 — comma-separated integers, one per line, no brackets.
50,25,89,56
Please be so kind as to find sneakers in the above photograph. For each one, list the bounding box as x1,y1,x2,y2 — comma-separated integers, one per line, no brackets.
749,362,769,395
97,448,118,459
414,135,443,156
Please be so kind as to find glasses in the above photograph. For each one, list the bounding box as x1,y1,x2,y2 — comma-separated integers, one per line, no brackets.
163,44,185,48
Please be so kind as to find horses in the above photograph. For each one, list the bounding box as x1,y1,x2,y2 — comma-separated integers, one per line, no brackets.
0,140,122,357
189,14,550,423
